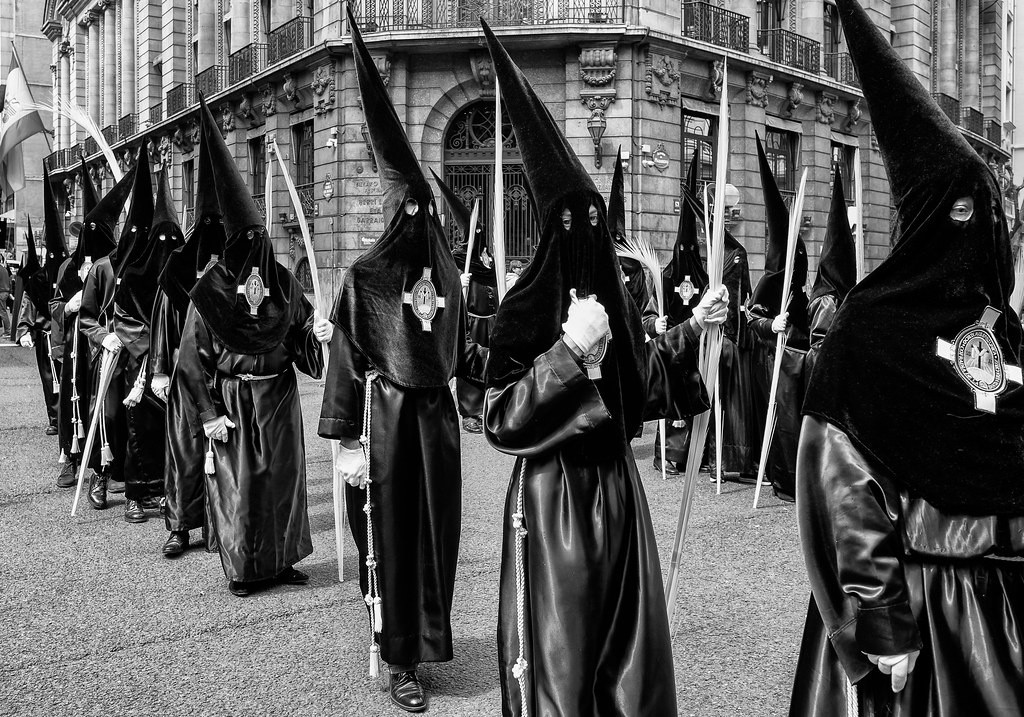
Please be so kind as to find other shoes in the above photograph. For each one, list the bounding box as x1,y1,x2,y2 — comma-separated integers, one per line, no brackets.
2,333,11,338
653,457,795,502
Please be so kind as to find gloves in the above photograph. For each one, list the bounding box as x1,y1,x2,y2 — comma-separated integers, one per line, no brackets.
460,273,472,288
20,332,34,348
692,284,729,331
655,316,668,335
771,312,789,333
69,290,235,443
313,308,333,343
336,443,367,489
562,288,613,353
862,650,919,692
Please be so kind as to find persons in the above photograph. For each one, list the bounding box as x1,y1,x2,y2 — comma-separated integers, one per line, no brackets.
10,204,333,598
320,185,463,712
428,217,524,434
0,253,23,338
481,176,729,717
787,155,1024,717
608,234,857,503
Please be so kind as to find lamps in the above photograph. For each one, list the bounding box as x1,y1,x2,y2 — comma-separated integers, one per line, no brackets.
587,109,606,169
361,120,377,172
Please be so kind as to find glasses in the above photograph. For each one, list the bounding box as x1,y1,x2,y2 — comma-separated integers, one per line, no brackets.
516,266,521,270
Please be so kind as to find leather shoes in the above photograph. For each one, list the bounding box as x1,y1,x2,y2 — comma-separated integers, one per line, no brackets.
47,425,190,553
463,418,481,434
229,580,248,594
281,568,309,582
388,664,428,711
